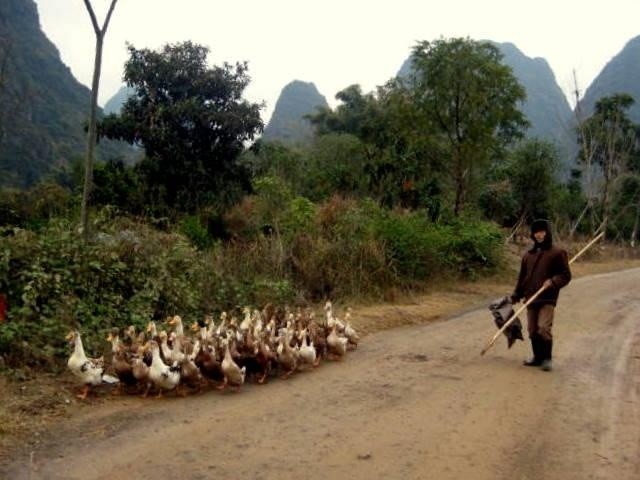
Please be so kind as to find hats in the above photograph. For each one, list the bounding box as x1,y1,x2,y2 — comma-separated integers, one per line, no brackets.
530,218,552,243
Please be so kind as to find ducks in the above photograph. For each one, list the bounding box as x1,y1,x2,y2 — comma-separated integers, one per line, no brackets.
64,327,106,401
106,300,360,400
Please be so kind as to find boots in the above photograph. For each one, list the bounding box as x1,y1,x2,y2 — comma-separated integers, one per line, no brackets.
524,336,541,366
540,339,552,370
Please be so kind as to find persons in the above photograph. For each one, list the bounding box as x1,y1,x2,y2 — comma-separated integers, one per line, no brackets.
505,219,572,372
